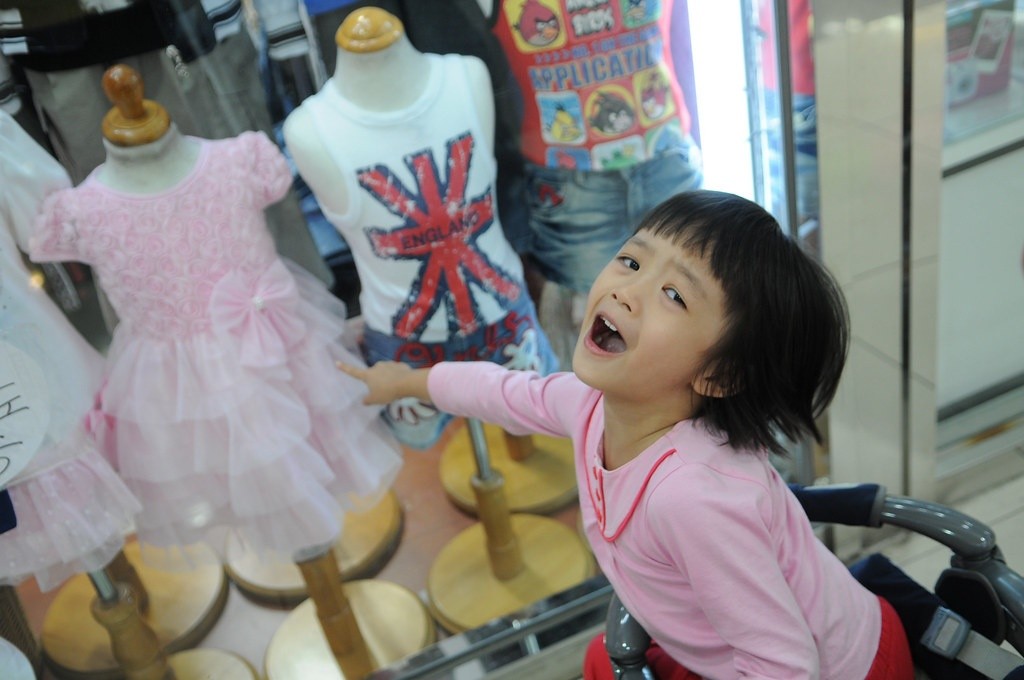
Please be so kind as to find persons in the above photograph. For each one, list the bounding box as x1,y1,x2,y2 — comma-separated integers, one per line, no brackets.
335,190,915,680
485,0,701,296
28,65,403,572
282,6,559,448
